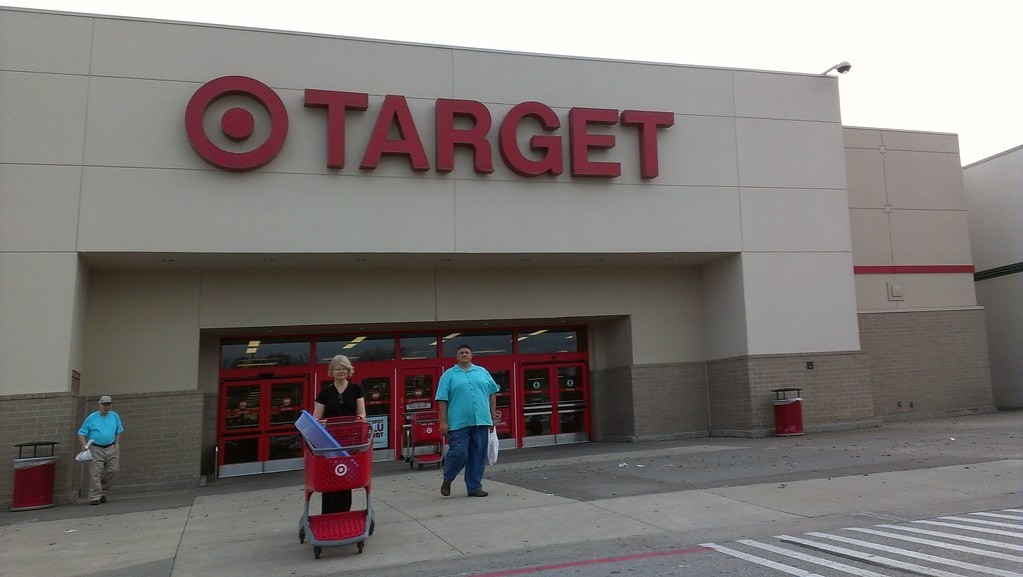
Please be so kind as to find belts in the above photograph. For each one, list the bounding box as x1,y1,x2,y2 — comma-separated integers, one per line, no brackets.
93,442,115,448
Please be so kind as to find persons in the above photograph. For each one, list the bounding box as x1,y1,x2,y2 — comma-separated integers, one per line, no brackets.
311,354,367,515
435,344,501,497
77,395,124,505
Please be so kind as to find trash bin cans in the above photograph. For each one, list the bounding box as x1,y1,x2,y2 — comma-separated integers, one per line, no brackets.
770,388,806,436
10,439,60,513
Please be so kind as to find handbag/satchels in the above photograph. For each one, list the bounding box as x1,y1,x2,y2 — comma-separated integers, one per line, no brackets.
488,426,500,465
75,440,94,462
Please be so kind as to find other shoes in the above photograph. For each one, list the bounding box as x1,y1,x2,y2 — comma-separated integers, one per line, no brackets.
90,500,98,505
441,480,450,496
100,496,106,503
468,489,488,497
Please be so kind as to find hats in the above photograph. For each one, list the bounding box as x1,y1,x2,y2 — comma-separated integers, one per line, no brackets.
99,396,112,403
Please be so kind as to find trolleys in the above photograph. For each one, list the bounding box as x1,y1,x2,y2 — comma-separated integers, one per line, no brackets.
400,410,445,470
302,415,376,559
